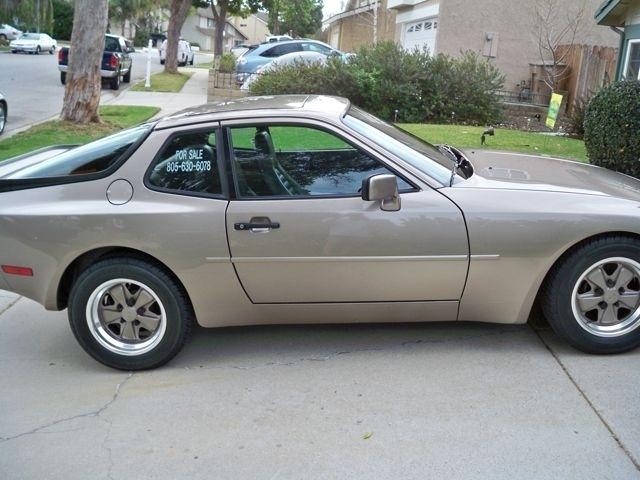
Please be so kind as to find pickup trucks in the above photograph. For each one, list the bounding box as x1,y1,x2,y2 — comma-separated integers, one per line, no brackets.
58,34,133,90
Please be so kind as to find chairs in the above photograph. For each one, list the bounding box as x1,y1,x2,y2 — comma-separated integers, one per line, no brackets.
151,131,312,197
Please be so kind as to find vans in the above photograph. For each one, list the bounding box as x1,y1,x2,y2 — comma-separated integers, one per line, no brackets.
158,39,195,68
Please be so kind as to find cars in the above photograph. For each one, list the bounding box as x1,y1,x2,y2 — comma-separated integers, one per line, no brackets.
0,23,59,55
0,94,640,373
229,34,359,93
